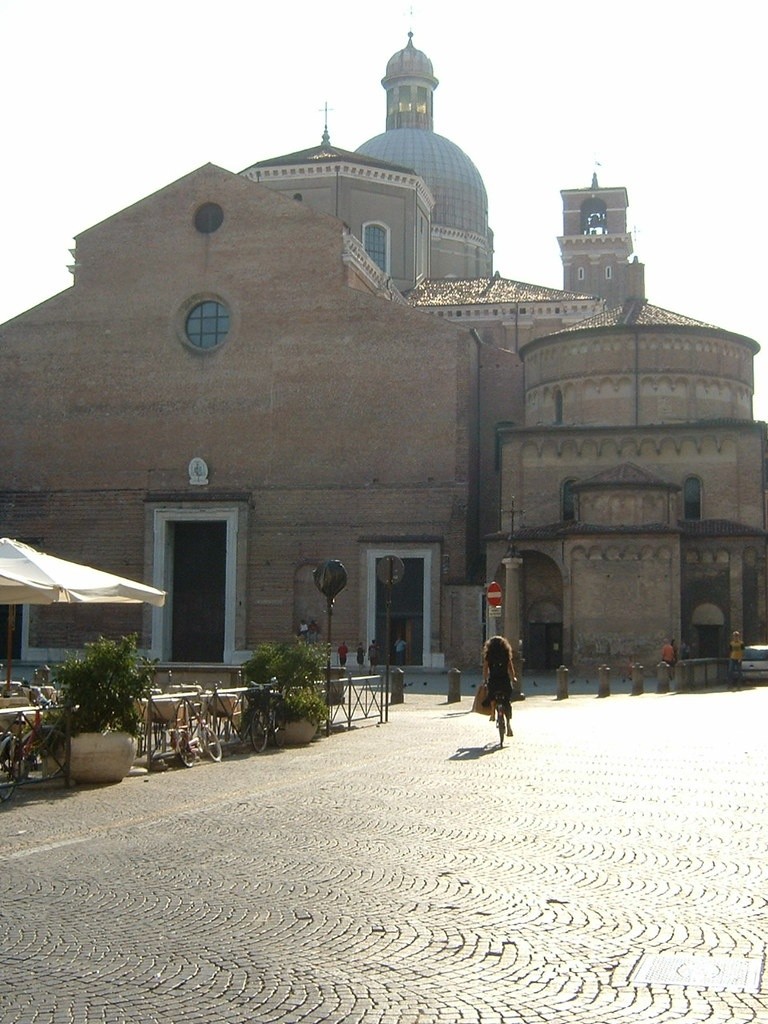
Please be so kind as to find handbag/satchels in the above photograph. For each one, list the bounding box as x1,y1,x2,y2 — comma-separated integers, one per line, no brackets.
472,682,492,714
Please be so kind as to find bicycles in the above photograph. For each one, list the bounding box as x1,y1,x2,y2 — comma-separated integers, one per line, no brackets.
176,701,224,768
0,676,71,806
249,681,287,753
484,678,516,748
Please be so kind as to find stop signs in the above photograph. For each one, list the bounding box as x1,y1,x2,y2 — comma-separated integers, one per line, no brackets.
487,581,503,606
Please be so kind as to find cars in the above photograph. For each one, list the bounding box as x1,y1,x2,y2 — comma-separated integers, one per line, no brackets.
731,643,768,682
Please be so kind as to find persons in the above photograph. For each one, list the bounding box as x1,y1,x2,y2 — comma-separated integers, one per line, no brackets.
367,640,379,675
393,636,408,666
483,636,517,737
338,641,348,668
306,620,320,645
356,642,366,676
661,638,690,681
296,620,308,639
727,631,745,691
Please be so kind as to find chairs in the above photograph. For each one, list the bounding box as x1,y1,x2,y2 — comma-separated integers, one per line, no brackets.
0,681,241,803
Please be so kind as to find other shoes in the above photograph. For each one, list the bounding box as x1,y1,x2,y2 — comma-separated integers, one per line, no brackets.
489,715,495,721
507,726,513,736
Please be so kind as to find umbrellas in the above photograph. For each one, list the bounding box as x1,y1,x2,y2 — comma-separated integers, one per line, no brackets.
0,537,165,696
